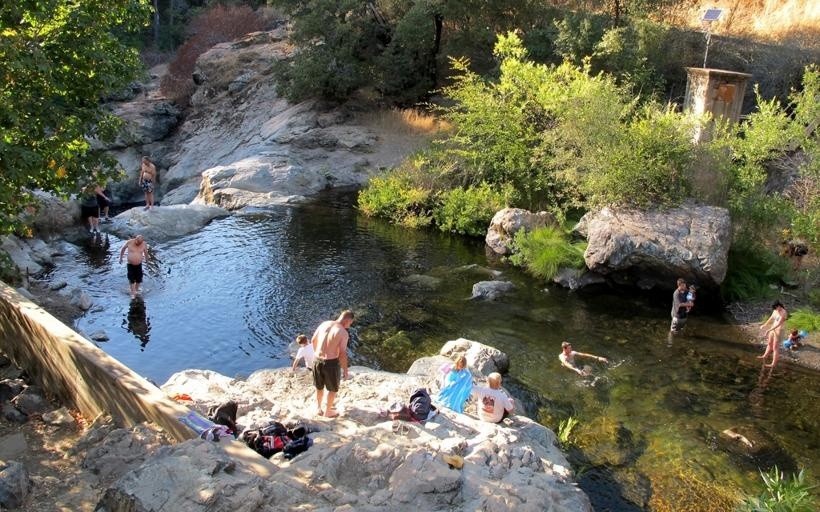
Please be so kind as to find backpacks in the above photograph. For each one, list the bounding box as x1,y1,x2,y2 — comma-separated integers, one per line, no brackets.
410,387,432,420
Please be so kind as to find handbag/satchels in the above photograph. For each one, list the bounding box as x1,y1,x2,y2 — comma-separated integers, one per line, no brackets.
244,422,312,461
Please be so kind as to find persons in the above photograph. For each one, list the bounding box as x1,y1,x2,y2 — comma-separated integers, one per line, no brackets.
311,310,354,417
81,178,111,233
119,235,150,300
583,365,592,379
559,341,608,377
755,300,787,368
472,371,514,424
138,155,156,209
667,278,689,348
789,329,803,349
686,284,696,314
291,334,315,372
438,356,471,412
93,165,113,222
